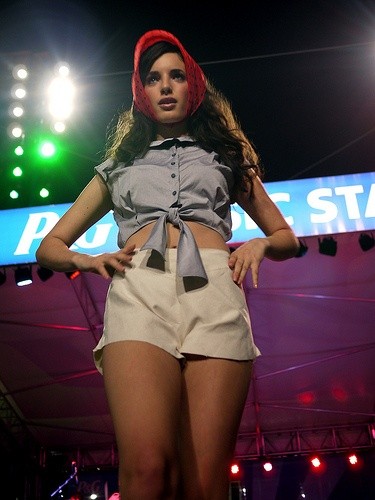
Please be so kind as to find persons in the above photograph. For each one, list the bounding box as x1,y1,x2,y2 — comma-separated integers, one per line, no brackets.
36,30,297,475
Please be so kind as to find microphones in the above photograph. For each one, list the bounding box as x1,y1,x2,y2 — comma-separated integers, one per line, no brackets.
72,462,78,484
